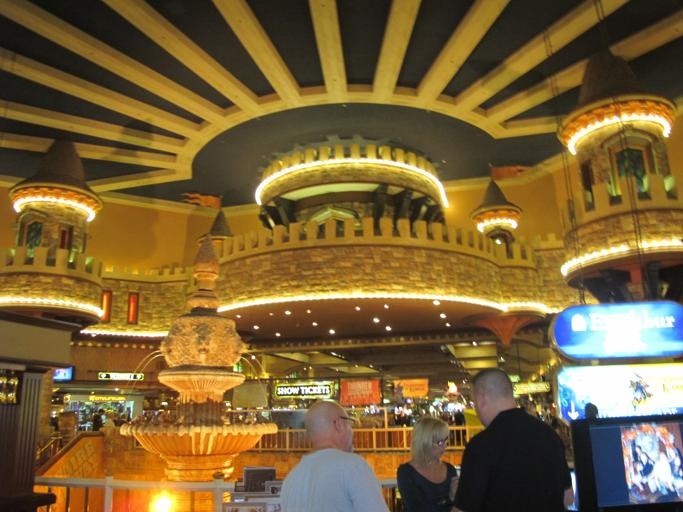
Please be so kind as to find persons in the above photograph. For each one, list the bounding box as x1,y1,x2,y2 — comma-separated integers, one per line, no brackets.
93,411,104,430
397,418,460,510
632,436,681,497
451,367,575,512
280,396,393,511
545,402,565,447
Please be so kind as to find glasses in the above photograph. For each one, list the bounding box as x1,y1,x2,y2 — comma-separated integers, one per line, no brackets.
434,437,448,446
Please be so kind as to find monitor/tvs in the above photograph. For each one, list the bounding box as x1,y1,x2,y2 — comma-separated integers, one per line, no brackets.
52,365,76,383
569,413,683,512
